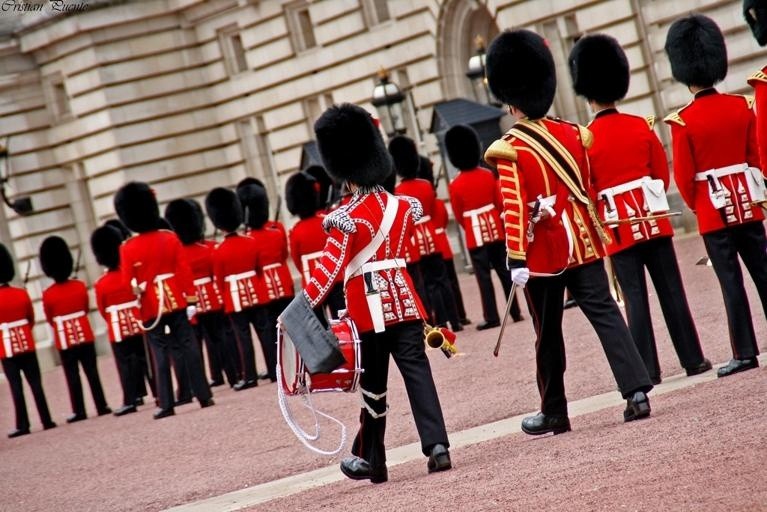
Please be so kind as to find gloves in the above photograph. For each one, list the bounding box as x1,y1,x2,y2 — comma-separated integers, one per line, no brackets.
511,268,529,289
186,306,196,320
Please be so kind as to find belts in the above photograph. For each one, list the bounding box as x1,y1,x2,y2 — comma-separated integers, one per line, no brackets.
224,270,257,282
52,310,86,323
463,203,495,218
435,228,444,234
695,163,751,182
350,258,407,278
193,277,212,285
528,195,557,211
414,215,431,226
154,272,174,283
597,175,654,201
105,300,140,313
301,251,324,261
263,263,282,270
0,319,28,330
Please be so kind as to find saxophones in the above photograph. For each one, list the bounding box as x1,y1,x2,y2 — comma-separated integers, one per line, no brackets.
422,322,458,359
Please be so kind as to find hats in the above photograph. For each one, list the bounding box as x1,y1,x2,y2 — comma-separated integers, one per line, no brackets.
314,103,393,187
114,181,160,232
39,236,73,280
105,219,130,241
486,28,557,121
91,225,121,269
160,198,205,245
742,0,767,45
445,124,481,171
305,166,330,203
665,15,728,87
568,34,630,105
418,156,433,181
389,136,419,179
236,177,269,229
206,188,243,233
0,243,14,284
286,171,319,219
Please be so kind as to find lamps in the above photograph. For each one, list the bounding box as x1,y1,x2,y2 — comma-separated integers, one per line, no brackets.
370,66,407,138
20,196,29,197
465,35,502,113
0,145,30,215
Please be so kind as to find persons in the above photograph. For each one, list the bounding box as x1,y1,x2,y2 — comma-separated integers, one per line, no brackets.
276,101,449,484
487,32,652,435
564,287,578,308
744,1,767,209
39,236,111,423
389,124,524,332
0,246,57,438
664,15,767,376
89,165,355,419
569,34,710,383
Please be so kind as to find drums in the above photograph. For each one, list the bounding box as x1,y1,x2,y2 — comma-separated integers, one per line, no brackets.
274,315,365,396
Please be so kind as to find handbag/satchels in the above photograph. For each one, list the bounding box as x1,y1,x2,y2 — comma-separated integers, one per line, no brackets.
743,167,767,205
641,179,670,214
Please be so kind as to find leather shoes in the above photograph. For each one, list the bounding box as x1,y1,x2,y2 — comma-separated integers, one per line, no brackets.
234,380,257,390
452,322,464,332
460,319,471,324
624,391,651,422
521,411,570,435
686,359,713,376
153,408,175,419
427,444,451,473
717,359,759,378
8,428,30,437
175,399,193,406
477,321,500,330
258,374,271,379
114,398,144,416
67,414,87,423
43,422,56,430
210,381,225,387
514,315,525,322
201,399,215,408
340,457,388,483
97,408,112,416
